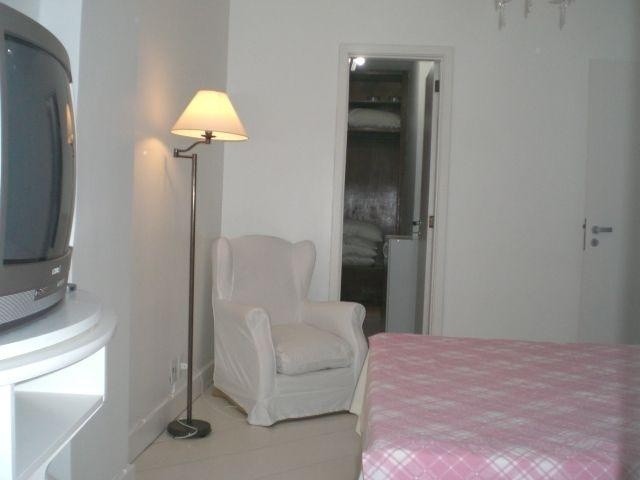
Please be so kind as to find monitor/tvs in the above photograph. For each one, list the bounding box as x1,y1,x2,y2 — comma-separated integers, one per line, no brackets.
0,1,78,334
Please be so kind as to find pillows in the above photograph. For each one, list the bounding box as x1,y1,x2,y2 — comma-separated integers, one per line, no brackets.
342,220,384,269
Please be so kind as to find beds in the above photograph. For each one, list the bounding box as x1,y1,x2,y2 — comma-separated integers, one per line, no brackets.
349,331,639,480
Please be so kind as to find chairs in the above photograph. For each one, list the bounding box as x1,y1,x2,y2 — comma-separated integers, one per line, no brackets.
208,232,368,426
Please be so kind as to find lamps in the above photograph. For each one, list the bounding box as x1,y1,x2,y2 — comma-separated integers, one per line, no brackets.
168,87,249,440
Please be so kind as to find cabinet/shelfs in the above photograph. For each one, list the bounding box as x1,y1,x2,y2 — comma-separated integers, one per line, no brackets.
0,287,116,479
342,70,410,304
